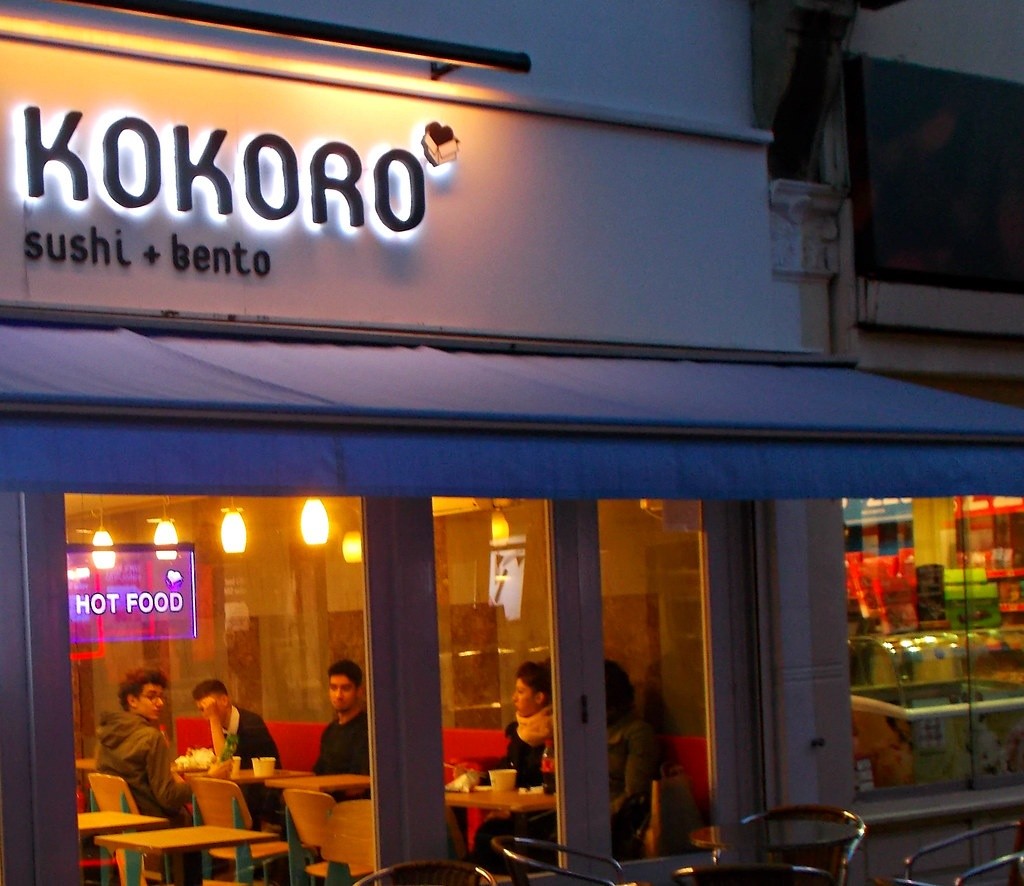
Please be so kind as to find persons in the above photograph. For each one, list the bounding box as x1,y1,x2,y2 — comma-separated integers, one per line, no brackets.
194,681,281,879
94,668,232,831
474,659,662,877
312,659,371,803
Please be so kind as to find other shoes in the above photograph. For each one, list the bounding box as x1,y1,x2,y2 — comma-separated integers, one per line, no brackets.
212,858,230,876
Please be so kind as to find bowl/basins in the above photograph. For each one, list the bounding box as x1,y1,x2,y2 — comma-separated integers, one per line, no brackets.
251,758,276,777
488,769,517,792
231,756,241,778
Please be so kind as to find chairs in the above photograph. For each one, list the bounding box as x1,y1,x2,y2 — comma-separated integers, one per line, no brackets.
87,773,1024,886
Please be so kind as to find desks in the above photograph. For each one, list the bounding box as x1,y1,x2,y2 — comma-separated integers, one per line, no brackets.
691,825,856,855
267,770,369,798
850,781,1024,830
93,825,280,886
77,811,168,834
193,765,315,786
445,784,558,818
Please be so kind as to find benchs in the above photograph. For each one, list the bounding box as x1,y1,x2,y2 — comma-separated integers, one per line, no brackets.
176,717,708,828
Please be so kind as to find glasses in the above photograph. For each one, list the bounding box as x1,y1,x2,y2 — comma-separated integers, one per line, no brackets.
329,683,352,690
141,693,167,700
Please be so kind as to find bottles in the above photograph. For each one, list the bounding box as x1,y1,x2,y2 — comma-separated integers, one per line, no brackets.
220,734,239,763
541,740,554,794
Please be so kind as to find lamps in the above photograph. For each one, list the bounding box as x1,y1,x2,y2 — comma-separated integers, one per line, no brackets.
147,498,179,560
300,495,330,545
91,494,116,569
219,496,247,554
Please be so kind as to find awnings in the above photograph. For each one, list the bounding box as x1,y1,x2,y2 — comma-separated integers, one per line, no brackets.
0,322,1024,500
